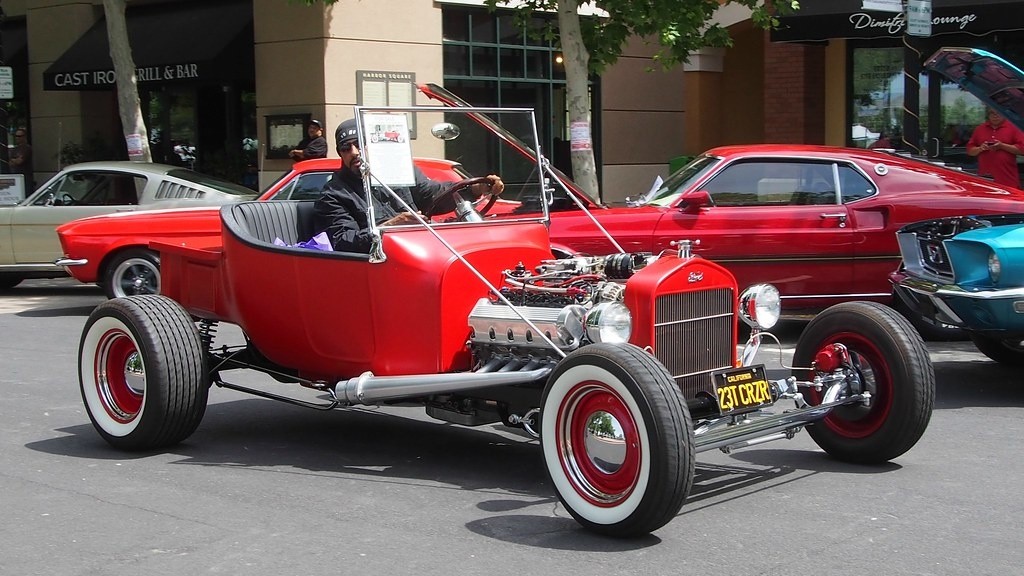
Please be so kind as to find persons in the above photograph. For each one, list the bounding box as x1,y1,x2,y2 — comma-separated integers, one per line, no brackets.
288,119,328,162
312,119,505,254
10,130,34,199
965,105,1024,190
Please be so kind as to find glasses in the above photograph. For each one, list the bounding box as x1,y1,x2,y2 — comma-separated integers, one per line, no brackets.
987,110,1002,117
338,136,366,152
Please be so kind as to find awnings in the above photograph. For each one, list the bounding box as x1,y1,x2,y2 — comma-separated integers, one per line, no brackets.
42,0,254,91
769,0,1024,46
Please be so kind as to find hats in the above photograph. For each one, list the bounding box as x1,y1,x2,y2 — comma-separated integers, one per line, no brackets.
335,119,366,145
308,119,323,128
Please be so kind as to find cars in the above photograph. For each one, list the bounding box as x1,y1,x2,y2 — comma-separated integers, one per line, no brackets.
408,82,1024,333
77,105,937,540
890,47,1024,364
0,161,259,292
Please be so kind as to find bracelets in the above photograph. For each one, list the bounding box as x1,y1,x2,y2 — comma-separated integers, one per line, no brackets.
294,150,296,155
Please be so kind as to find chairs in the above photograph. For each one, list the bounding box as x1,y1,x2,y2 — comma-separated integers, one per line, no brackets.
805,183,835,204
295,201,317,246
